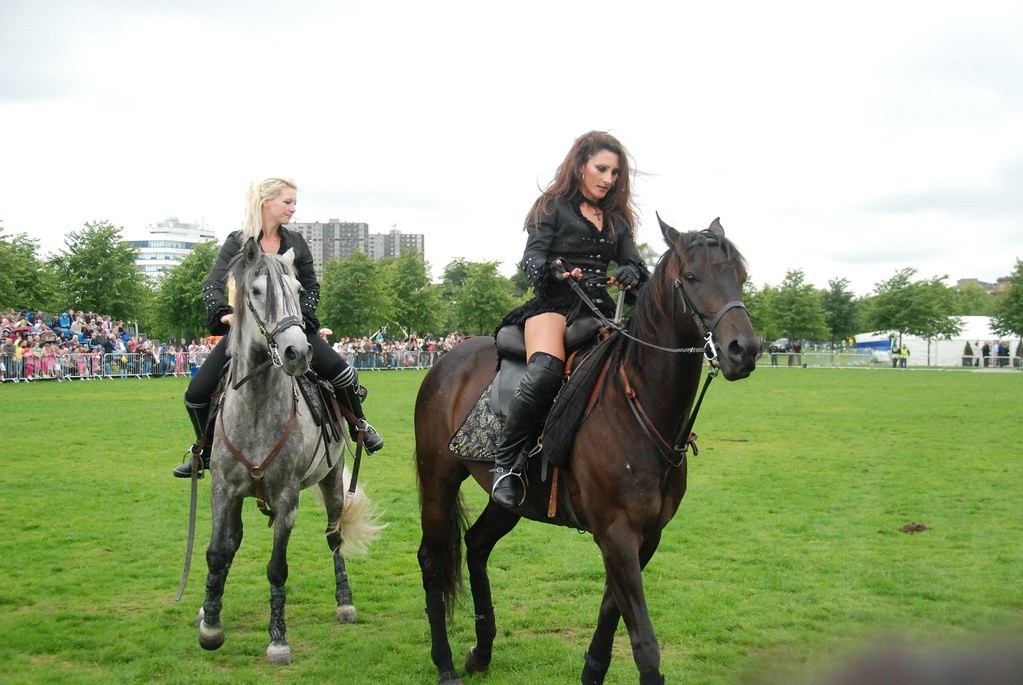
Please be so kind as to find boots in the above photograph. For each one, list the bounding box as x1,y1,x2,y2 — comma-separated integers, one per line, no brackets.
334,366,384,453
173,388,212,477
491,352,565,509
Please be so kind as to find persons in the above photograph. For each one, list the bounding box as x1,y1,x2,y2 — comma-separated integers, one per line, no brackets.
974,341,1009,367
492,131,651,515
321,335,328,343
841,336,853,351
768,341,778,365
135,337,218,379
0,308,135,381
899,344,911,368
338,333,465,370
786,339,803,366
892,344,900,367
173,172,383,479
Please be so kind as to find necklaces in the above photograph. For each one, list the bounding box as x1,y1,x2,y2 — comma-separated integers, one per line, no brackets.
578,189,603,222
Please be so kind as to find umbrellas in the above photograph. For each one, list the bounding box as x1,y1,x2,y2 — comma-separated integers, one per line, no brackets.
58,341,75,349
319,328,333,334
39,340,56,348
12,326,33,333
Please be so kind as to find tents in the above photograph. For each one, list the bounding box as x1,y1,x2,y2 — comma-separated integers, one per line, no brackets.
894,315,1022,368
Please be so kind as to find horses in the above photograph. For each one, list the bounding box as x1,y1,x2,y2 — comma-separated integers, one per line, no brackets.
413,207,764,685
196,234,366,667
